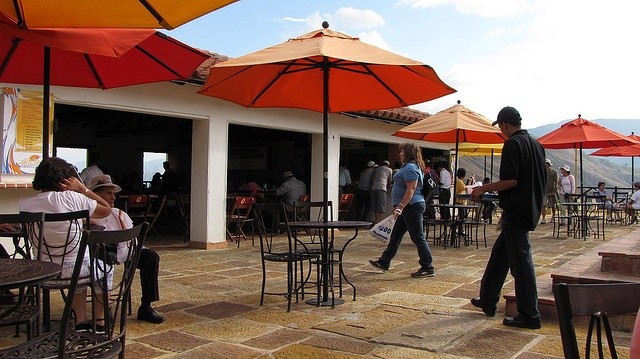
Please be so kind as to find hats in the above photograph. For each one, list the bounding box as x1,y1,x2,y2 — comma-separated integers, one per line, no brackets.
559,165,570,171
545,158,553,166
89,175,122,193
492,106,522,126
283,172,293,177
368,161,379,167
382,161,390,165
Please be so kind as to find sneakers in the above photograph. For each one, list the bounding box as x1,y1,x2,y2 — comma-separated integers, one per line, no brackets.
369,257,390,271
96,320,105,334
137,304,166,324
560,220,566,226
541,219,546,224
75,322,91,332
550,218,555,222
411,267,435,278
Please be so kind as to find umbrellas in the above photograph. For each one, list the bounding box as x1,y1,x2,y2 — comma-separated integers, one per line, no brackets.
457,152,502,179
391,100,509,248
537,114,640,238
196,21,457,305
0,0,242,332
1,27,213,158
451,141,506,184
587,130,640,188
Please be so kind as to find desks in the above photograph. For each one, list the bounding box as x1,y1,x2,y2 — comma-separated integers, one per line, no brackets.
0,252,63,340
560,200,602,239
282,217,375,302
548,193,578,239
430,200,479,248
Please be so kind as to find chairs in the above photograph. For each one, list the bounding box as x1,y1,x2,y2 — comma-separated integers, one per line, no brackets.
4,222,150,359
292,201,343,299
33,207,115,334
592,188,614,225
465,197,489,250
1,213,52,340
225,194,254,242
552,278,640,358
423,194,451,247
126,190,168,243
340,192,354,220
612,191,629,227
250,201,327,312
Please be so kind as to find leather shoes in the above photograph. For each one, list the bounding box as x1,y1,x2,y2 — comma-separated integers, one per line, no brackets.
503,314,542,329
471,297,495,317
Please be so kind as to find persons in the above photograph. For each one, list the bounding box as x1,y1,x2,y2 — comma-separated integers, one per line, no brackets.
239,176,262,202
595,182,621,220
357,161,379,221
471,105,547,329
480,177,497,223
625,182,640,224
87,175,166,323
369,142,435,278
339,162,352,195
438,158,451,221
421,159,440,219
80,152,104,186
456,168,470,235
152,161,181,187
465,177,476,185
541,159,575,226
370,161,392,229
388,161,401,191
18,157,112,334
276,171,307,234
